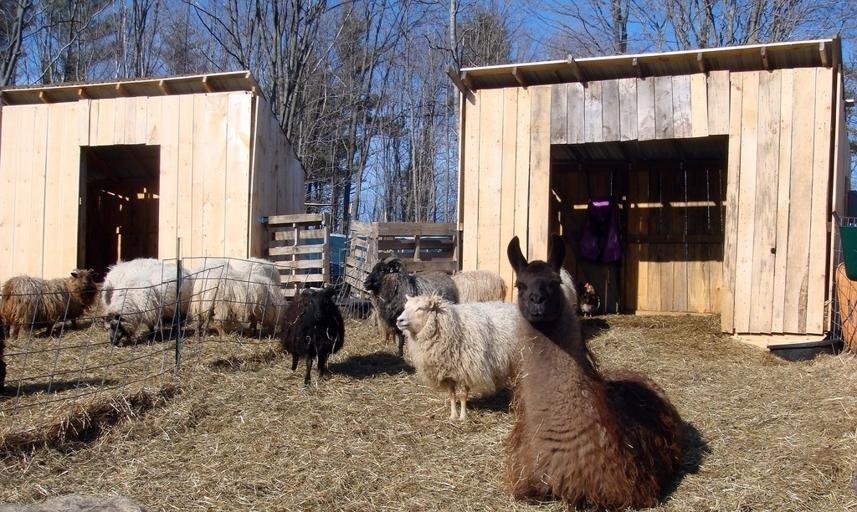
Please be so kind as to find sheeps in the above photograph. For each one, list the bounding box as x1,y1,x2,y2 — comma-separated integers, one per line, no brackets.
361,254,506,360
280,286,345,387
98,250,285,348
395,288,520,423
0,268,97,339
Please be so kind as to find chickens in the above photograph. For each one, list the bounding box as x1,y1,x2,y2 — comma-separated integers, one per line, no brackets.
580,282,600,317
